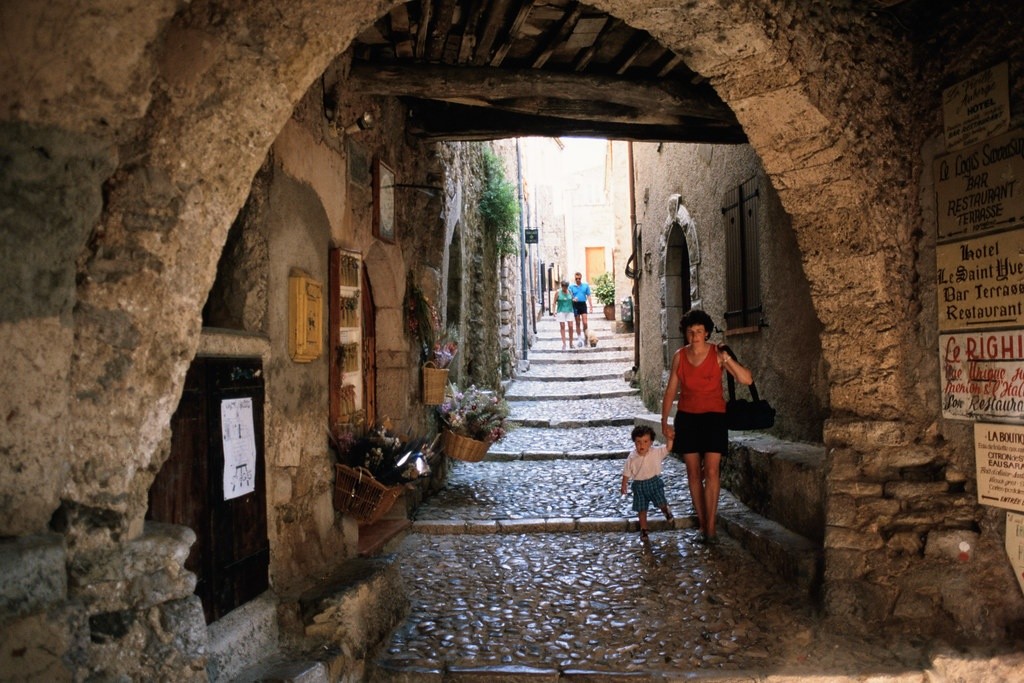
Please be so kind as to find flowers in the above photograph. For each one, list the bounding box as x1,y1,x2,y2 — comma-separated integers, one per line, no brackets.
322,323,513,489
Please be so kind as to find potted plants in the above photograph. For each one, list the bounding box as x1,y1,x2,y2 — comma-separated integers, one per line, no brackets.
595,272,617,321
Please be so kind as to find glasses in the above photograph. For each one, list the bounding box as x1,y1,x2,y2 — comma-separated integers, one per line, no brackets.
575,278,582,281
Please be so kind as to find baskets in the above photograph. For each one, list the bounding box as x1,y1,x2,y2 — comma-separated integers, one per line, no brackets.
438,425,496,462
421,360,457,408
334,465,403,524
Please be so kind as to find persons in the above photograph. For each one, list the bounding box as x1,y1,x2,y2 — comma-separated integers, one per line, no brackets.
662,310,753,546
620,425,675,542
568,272,593,347
553,279,578,350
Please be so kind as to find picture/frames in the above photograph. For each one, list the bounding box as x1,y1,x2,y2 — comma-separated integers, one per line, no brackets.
371,154,398,244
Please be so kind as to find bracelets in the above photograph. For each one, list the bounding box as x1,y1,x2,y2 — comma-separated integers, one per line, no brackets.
662,418,668,422
724,356,732,363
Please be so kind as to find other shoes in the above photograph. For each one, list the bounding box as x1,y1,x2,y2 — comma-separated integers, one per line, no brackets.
578,341,582,347
562,344,566,350
569,343,575,349
584,339,589,347
693,533,707,543
707,536,720,544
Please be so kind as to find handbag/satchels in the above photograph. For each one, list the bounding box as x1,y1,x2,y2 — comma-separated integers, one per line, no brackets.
721,346,781,431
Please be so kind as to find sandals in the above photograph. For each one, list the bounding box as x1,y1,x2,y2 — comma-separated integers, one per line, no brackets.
666,515,677,528
639,528,650,542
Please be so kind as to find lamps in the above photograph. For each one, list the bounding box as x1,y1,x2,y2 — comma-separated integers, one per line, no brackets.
346,112,376,134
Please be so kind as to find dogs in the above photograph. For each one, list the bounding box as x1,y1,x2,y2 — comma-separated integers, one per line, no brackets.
585,329,598,347
